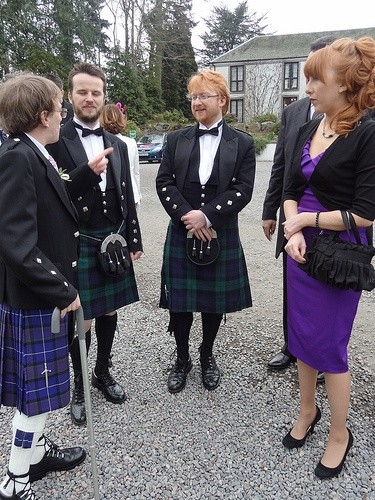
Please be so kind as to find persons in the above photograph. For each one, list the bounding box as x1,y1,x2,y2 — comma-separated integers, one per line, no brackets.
155,70,256,394
282,36,375,479
262,35,342,385
99,102,143,209
45,64,144,426
0,76,114,500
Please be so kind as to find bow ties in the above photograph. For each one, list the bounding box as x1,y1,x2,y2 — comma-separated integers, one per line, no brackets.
195,119,224,138
72,120,104,138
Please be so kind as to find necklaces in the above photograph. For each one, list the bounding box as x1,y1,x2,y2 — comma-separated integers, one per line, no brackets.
323,118,335,138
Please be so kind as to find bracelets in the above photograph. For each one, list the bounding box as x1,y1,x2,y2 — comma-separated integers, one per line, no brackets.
316,210,321,229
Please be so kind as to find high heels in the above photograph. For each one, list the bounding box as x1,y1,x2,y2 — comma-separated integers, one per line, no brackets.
314,426,354,478
282,405,321,449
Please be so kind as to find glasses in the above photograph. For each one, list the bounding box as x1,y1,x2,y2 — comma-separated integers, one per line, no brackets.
48,107,69,118
188,93,218,102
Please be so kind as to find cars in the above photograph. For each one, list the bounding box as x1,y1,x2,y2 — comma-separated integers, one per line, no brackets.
136,132,169,164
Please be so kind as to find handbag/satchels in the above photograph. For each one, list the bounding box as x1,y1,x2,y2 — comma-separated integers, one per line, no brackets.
185,225,221,266
97,231,133,279
297,208,375,293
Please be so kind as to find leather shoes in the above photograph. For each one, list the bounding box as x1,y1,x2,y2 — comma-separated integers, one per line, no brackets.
317,372,325,383
70,387,86,425
0,479,41,500
29,443,87,483
200,352,222,391
267,351,297,370
168,352,193,393
91,367,127,404
